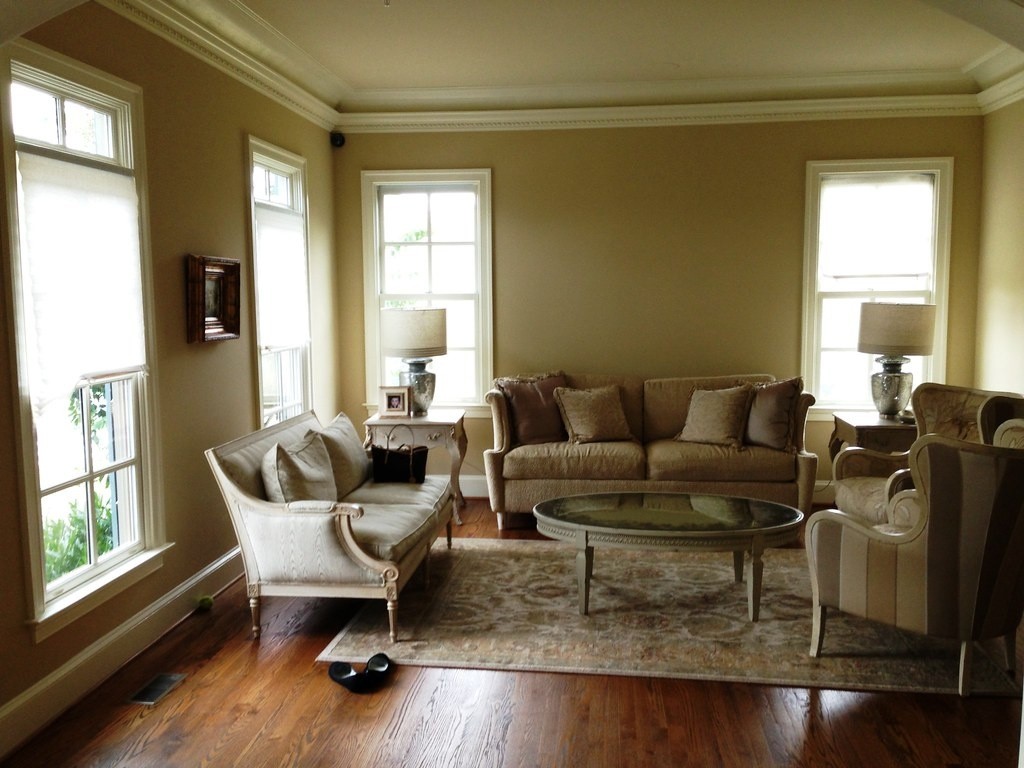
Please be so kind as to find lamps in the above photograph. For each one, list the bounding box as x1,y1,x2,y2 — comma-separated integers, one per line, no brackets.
857,303,937,417
381,308,447,417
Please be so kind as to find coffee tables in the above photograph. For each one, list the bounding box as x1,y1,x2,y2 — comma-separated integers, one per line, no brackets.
531,490,805,622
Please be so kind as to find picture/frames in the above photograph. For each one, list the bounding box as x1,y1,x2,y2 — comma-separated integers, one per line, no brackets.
378,385,412,419
186,253,241,344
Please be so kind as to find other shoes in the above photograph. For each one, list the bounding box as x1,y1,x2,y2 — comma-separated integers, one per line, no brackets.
328,661,372,693
366,653,396,688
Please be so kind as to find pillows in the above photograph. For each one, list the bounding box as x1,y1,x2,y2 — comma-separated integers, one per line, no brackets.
302,411,371,499
671,381,757,452
517,373,645,441
489,367,572,447
743,377,804,453
644,374,776,441
260,431,337,503
552,385,633,443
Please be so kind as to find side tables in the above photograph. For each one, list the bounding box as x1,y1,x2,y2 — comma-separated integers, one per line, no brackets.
364,407,467,526
827,412,917,466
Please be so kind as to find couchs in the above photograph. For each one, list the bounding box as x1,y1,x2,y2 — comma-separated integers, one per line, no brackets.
204,409,455,644
482,387,819,531
832,383,1024,523
803,418,1024,696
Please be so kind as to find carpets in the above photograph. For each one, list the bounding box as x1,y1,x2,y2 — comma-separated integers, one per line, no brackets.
315,537,1022,697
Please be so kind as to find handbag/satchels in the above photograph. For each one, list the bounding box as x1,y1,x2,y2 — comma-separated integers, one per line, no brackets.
372,424,428,484
